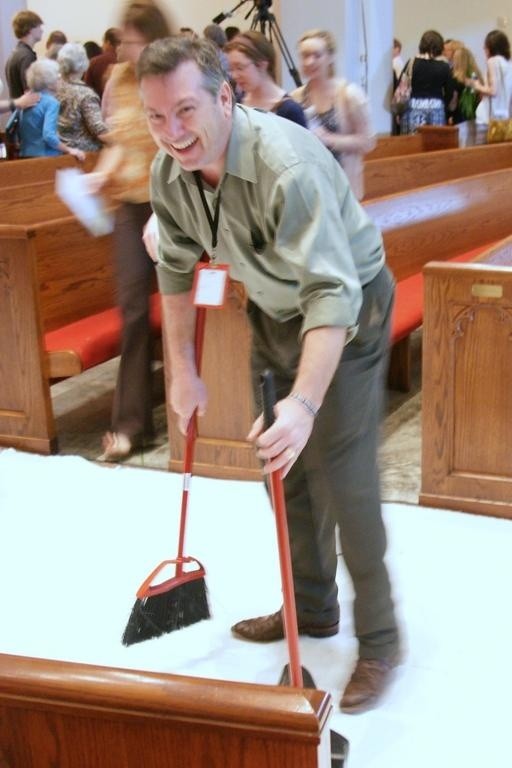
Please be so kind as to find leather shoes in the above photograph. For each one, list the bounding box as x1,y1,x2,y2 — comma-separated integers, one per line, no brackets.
340,648,404,713
232,610,341,644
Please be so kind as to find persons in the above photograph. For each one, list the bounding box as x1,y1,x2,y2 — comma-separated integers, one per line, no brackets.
89,1,173,464
134,34,407,714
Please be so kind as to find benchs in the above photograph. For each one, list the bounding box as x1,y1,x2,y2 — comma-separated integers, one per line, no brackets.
360,126,512,521
0,151,160,457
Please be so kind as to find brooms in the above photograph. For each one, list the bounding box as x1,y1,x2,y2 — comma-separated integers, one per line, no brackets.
122,308,213,651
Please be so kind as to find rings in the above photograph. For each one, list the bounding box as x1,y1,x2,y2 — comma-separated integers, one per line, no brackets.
283,449,297,460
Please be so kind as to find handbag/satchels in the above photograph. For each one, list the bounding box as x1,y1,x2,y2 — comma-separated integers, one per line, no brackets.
488,117,512,143
391,56,415,113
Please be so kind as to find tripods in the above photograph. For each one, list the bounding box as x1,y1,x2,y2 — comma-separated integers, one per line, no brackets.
249,14,302,88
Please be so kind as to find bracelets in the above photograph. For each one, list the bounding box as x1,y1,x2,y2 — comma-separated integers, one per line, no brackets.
287,391,318,419
